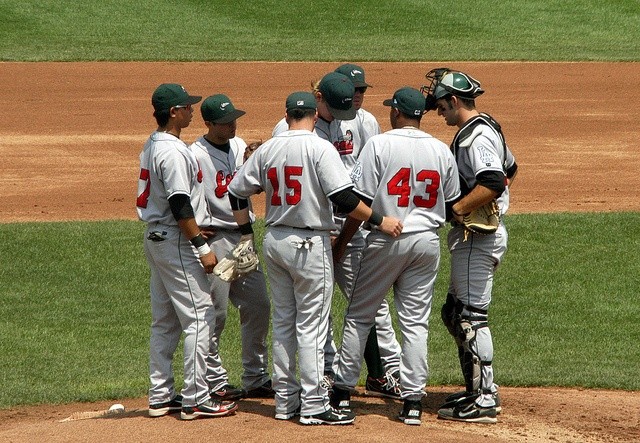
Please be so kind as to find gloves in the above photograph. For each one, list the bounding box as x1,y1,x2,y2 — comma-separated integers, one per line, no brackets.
292,237,314,250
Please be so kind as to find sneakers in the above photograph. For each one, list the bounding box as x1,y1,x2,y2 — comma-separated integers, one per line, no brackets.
445,389,502,413
275,404,302,420
437,395,502,424
242,380,276,398
364,370,401,400
399,399,422,425
324,372,351,413
300,407,355,425
209,385,244,402
181,398,238,420
149,395,183,416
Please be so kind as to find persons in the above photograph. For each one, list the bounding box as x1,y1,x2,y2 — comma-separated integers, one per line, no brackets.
226,91,404,424
329,86,461,424
271,71,357,387
334,63,428,382
136,82,239,419
434,72,518,424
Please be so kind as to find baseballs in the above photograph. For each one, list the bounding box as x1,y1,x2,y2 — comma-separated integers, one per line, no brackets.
108,403,125,411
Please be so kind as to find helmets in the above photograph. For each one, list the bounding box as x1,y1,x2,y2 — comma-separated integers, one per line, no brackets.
419,67,485,115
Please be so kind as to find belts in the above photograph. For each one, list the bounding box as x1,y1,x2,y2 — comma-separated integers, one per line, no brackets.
275,224,314,230
217,228,242,231
451,222,465,228
332,205,346,215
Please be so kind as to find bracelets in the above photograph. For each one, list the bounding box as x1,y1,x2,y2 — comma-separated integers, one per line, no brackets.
238,220,254,235
367,207,383,226
451,207,464,215
187,92,277,399
197,241,211,256
190,231,206,247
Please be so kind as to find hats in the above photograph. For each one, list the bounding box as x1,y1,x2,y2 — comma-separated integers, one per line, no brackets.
335,63,373,88
286,92,317,112
320,72,356,120
152,83,202,111
201,94,246,124
383,87,426,116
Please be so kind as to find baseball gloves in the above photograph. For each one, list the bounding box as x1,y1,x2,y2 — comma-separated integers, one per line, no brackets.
243,138,264,195
456,197,501,242
212,238,260,282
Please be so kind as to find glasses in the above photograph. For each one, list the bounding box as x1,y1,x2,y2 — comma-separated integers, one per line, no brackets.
354,87,366,93
174,104,191,110
